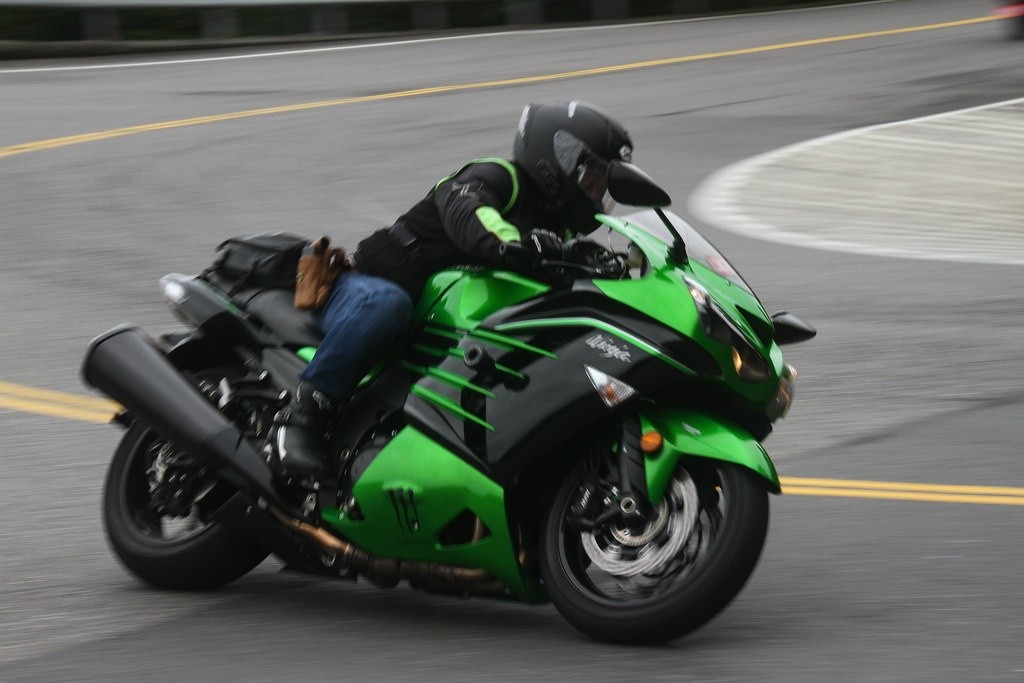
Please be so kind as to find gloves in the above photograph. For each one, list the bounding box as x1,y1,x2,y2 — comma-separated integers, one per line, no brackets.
521,228,622,287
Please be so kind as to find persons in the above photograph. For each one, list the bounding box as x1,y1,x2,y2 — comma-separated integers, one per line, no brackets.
267,99,632,472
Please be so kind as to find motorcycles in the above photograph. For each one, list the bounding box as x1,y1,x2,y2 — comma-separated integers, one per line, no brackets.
81,157,816,649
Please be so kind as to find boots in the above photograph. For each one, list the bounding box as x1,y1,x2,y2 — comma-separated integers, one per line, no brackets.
276,379,350,477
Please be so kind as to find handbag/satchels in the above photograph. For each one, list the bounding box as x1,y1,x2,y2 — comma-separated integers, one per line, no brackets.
213,232,306,286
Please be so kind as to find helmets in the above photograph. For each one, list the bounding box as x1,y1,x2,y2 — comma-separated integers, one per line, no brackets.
513,99,633,237
